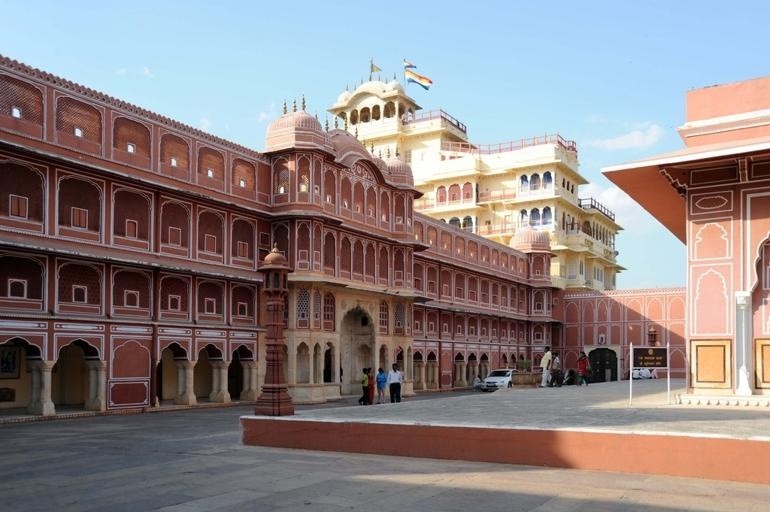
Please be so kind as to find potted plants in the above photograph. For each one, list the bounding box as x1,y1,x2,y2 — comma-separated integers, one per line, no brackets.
511,355,541,388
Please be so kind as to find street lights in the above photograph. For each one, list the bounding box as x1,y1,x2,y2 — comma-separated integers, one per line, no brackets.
255,240,296,417
647,324,657,347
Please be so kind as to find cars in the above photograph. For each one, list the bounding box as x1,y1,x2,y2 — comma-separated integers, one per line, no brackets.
480,368,519,394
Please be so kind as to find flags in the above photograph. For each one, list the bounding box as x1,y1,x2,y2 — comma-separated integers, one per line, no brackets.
372,64,382,72
405,70,433,91
404,60,416,69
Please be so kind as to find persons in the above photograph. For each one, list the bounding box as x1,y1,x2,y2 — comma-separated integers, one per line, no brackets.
376,367,387,403
368,367,375,403
632,368,658,379
359,368,369,404
473,376,481,390
388,363,402,402
576,351,591,386
540,346,556,387
548,352,563,387
